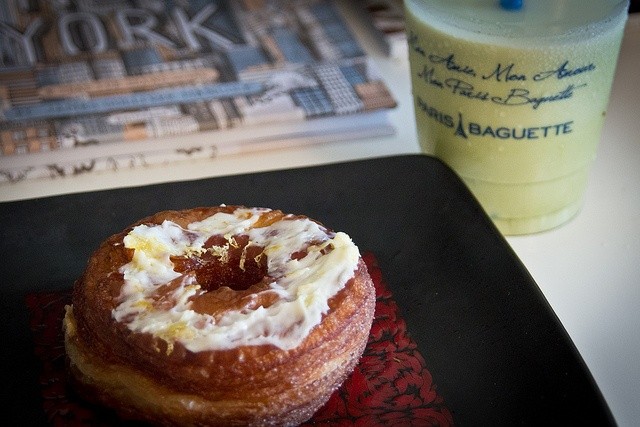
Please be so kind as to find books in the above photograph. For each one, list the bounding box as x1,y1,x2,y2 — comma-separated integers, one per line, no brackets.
0,1,399,189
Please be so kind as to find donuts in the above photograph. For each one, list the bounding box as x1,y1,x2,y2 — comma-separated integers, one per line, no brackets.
60,205,376,427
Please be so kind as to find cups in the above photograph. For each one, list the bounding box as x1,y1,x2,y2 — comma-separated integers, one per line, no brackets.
402,2,630,235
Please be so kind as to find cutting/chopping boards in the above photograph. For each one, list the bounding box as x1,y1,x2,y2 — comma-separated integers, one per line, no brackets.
2,153,619,425
